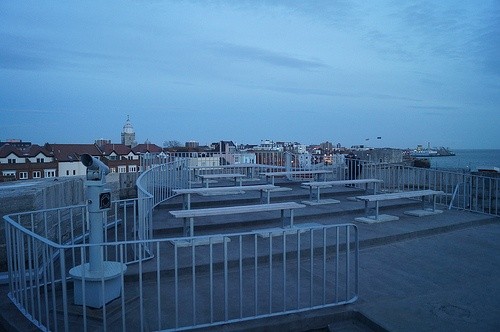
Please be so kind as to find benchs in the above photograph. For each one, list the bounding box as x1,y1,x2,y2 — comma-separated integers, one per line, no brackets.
301,180,384,205
260,171,333,188
355,189,444,224
199,174,247,196
169,202,324,247
173,184,292,209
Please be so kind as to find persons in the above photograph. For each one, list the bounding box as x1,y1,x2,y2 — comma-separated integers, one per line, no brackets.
345,152,362,188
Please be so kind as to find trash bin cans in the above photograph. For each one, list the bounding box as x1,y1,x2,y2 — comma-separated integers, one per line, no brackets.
468,166,500,211
120,187,139,207
